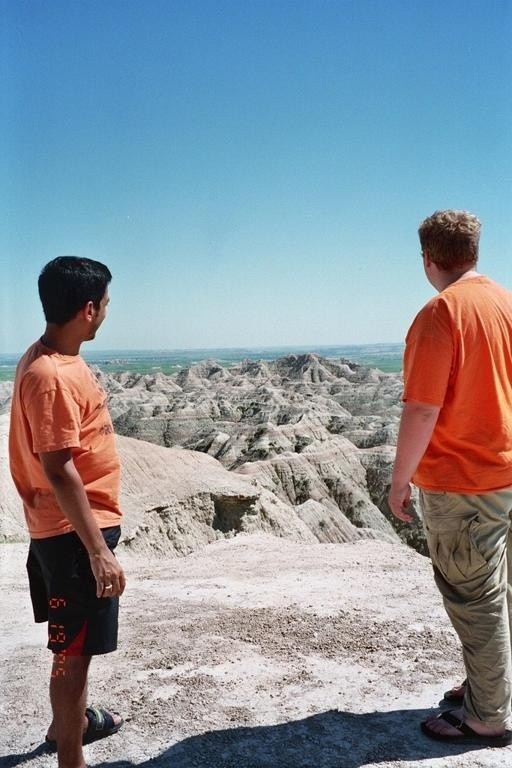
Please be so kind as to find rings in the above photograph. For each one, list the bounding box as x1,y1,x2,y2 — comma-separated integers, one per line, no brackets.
105,584,113,590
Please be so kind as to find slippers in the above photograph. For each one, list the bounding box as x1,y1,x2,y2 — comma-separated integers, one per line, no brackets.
443,680,467,703
420,709,512,748
45,706,124,753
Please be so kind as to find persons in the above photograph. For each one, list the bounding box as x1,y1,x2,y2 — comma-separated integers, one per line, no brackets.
387,211,511,745
10,256,127,767
